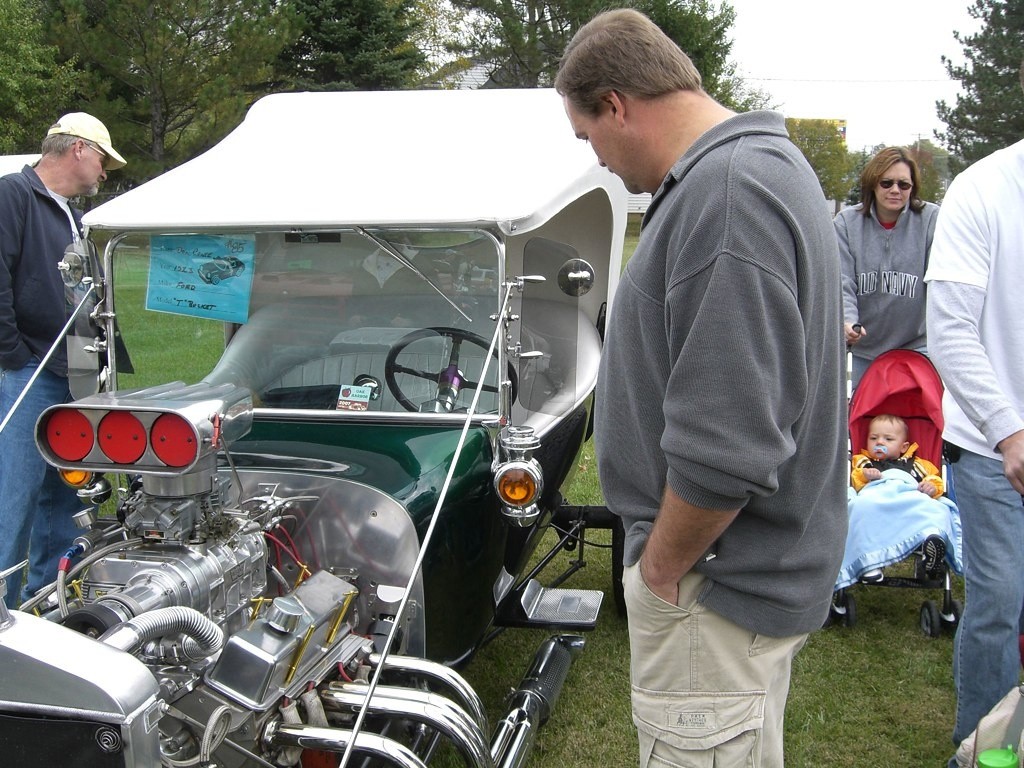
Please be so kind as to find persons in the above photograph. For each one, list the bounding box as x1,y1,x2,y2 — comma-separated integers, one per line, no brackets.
832,147,940,394
851,415,944,585
948,685,1024,768
924,138,1024,748
0,113,135,609
348,232,443,328
555,9,848,767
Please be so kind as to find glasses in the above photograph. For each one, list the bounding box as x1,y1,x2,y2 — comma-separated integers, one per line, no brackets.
878,178,914,190
72,140,110,171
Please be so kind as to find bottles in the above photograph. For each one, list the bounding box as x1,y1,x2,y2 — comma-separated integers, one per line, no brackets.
977,744,1019,768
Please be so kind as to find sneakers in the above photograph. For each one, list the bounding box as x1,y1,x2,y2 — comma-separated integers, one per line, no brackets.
861,568,884,584
921,534,946,581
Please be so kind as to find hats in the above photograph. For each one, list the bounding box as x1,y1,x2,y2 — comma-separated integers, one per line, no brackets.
48,112,127,170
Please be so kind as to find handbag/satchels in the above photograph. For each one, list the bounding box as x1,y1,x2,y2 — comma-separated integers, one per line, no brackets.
956,683,1024,768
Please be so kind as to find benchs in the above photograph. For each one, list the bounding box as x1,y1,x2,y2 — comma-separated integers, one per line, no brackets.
207,294,602,448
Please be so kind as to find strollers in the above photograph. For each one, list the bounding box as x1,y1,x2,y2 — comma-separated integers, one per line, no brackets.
823,319,962,637
